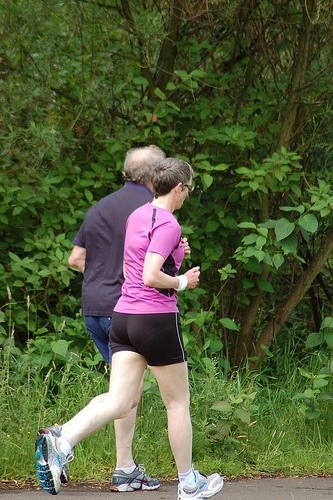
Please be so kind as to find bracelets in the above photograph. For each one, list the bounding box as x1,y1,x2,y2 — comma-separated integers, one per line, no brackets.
174,275,188,292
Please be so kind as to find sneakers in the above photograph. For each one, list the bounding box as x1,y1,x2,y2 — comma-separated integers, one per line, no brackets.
33,434,67,495
109,462,160,492
36,422,69,484
176,468,224,500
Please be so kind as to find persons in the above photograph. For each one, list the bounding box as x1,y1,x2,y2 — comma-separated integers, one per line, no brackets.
37,144,167,493
35,157,225,500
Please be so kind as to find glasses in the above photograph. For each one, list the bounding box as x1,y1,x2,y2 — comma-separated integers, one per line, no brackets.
184,184,194,192
